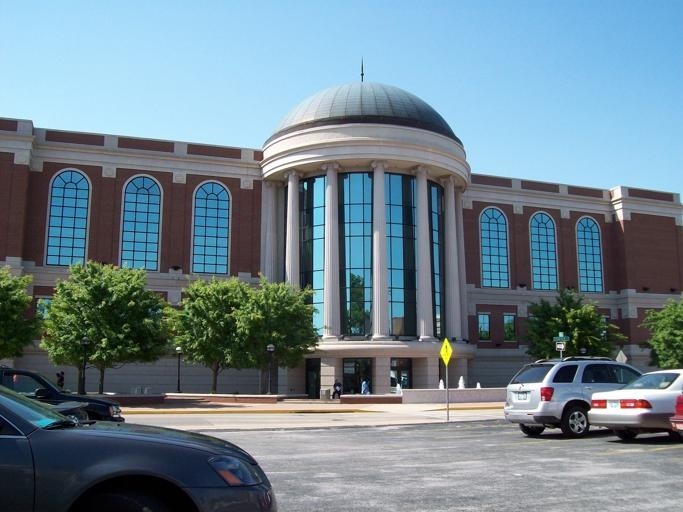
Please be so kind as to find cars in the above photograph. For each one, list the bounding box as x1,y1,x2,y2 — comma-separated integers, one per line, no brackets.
504,356,683,442
0,385,278,512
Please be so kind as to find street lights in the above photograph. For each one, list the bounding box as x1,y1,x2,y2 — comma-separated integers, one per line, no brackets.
81,337,89,395
265,344,275,395
175,347,183,393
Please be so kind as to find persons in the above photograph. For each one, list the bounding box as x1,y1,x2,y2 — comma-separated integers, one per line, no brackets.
332,379,342,399
55,371,64,389
361,377,369,394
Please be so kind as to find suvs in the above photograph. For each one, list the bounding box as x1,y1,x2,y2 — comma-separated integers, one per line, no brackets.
0,364,126,423
